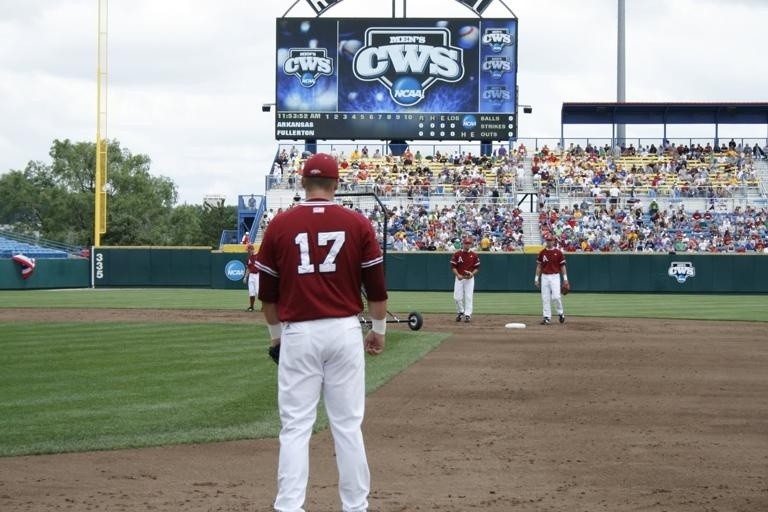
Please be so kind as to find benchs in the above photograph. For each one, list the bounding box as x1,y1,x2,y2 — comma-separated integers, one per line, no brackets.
252,147,768,253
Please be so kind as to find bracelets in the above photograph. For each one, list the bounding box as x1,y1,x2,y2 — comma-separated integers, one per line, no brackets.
370,319,387,335
563,274,568,281
535,276,539,281
267,323,281,340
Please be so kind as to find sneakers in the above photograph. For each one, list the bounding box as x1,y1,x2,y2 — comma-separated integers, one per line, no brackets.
455,312,464,322
244,306,256,312
540,316,552,325
559,311,565,324
464,315,471,323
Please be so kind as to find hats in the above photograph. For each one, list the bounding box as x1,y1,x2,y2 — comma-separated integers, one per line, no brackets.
463,237,473,245
245,245,255,251
246,232,249,234
301,153,338,178
543,232,556,241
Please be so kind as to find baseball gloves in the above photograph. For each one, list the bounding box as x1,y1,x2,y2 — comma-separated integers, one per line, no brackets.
562,280,570,296
462,270,473,279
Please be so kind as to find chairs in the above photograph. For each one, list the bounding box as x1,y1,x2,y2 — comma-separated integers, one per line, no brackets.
1,232,73,265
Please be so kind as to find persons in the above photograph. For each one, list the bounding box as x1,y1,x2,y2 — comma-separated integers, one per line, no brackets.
532,138,768,254
243,245,263,312
533,234,570,324
449,237,480,322
242,232,250,244
262,142,528,252
255,151,388,511
248,193,256,210
